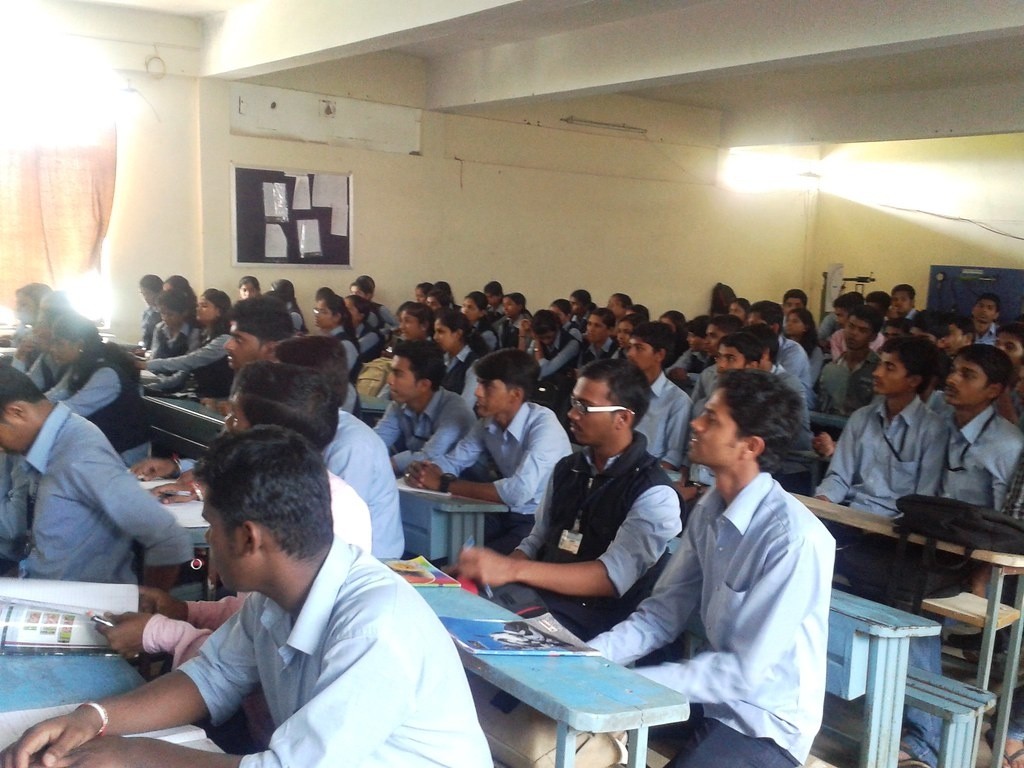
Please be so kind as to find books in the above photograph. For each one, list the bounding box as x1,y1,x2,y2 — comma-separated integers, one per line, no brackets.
0,702,226,754
383,555,461,588
440,612,602,656
0,576,139,657
128,468,212,528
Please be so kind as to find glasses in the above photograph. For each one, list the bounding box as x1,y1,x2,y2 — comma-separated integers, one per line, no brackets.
570,395,635,415
313,308,329,317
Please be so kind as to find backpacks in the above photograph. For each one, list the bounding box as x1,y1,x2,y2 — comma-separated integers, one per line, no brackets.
355,356,395,401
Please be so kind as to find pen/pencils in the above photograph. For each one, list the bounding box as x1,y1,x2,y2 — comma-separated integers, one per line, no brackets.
172,454,203,502
92,616,113,626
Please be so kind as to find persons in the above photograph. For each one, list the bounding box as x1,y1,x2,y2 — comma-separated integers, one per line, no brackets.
0,275,1024,767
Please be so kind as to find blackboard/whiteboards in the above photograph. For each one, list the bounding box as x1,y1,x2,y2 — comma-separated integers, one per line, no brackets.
229,161,355,271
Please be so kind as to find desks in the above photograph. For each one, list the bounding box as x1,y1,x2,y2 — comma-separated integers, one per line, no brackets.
379,558,690,768
788,492,1024,768
359,393,391,413
825,588,941,768
398,477,509,564
142,396,227,461
0,653,148,716
809,410,849,428
137,527,216,683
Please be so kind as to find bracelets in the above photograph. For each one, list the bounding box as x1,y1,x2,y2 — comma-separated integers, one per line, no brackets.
76,702,110,737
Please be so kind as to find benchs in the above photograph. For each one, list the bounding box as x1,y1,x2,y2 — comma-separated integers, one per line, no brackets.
905,664,997,768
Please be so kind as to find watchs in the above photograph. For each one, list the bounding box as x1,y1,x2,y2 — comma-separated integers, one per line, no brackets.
440,472,456,492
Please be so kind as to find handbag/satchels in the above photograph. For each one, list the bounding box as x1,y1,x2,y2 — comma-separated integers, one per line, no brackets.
893,494,1024,555
